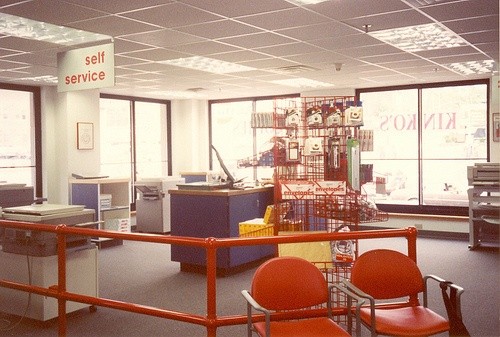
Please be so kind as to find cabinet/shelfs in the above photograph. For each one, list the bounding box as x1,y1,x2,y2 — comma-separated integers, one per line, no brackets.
69,178,131,249
239,95,389,331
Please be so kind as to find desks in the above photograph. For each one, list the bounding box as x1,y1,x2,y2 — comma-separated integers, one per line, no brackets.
168,183,274,277
468,188,500,250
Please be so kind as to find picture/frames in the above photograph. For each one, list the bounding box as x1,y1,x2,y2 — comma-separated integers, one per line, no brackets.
77,122,94,150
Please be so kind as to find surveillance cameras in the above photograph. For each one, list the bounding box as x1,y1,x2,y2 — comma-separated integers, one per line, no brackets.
336,64,343,71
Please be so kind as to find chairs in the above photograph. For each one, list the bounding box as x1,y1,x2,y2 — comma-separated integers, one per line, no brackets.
241,256,365,337
343,249,464,337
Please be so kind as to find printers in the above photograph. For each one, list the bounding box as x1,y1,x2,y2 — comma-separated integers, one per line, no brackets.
0,203,95,246
